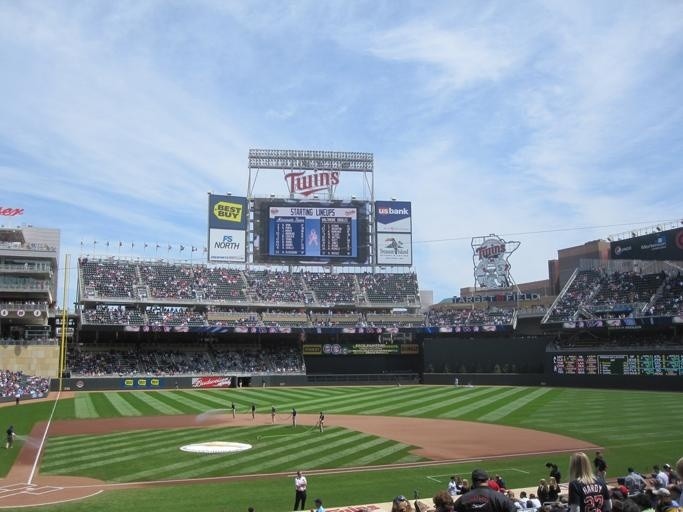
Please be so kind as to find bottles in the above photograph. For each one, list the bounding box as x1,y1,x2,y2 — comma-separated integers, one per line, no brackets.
663,464,671,469
313,499,321,504
650,487,671,496
472,468,490,482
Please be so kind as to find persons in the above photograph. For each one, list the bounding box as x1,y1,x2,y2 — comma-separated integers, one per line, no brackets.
392,450,683,512
232,403,235,419
0,369,49,405
319,412,324,433
272,407,275,424
67,257,683,378
294,471,307,511
0,297,59,346
5,425,15,449
252,405,255,418
311,498,324,512
248,506,255,512
292,408,296,427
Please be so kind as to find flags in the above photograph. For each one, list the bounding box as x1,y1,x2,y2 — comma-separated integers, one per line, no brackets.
81,240,208,253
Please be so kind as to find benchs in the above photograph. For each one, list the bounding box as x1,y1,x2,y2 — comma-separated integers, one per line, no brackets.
81,240,208,253
